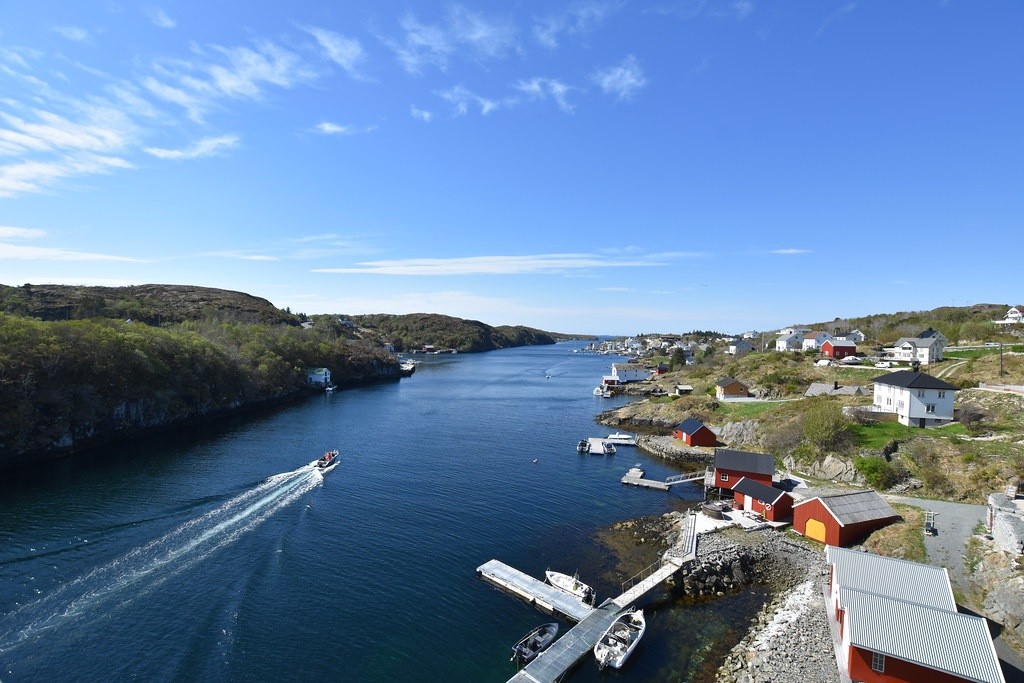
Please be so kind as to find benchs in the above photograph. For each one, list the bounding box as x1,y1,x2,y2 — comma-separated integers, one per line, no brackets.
742,512,757,523
760,514,764,521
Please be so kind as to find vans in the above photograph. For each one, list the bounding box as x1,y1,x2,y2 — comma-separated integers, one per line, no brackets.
874,361,893,369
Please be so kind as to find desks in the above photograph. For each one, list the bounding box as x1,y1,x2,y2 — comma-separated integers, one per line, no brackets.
745,509,762,523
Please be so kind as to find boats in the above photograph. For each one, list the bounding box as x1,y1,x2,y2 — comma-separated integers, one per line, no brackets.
509,622,560,664
593,386,614,399
576,439,590,453
317,449,340,467
593,608,648,671
603,440,617,455
544,569,595,607
